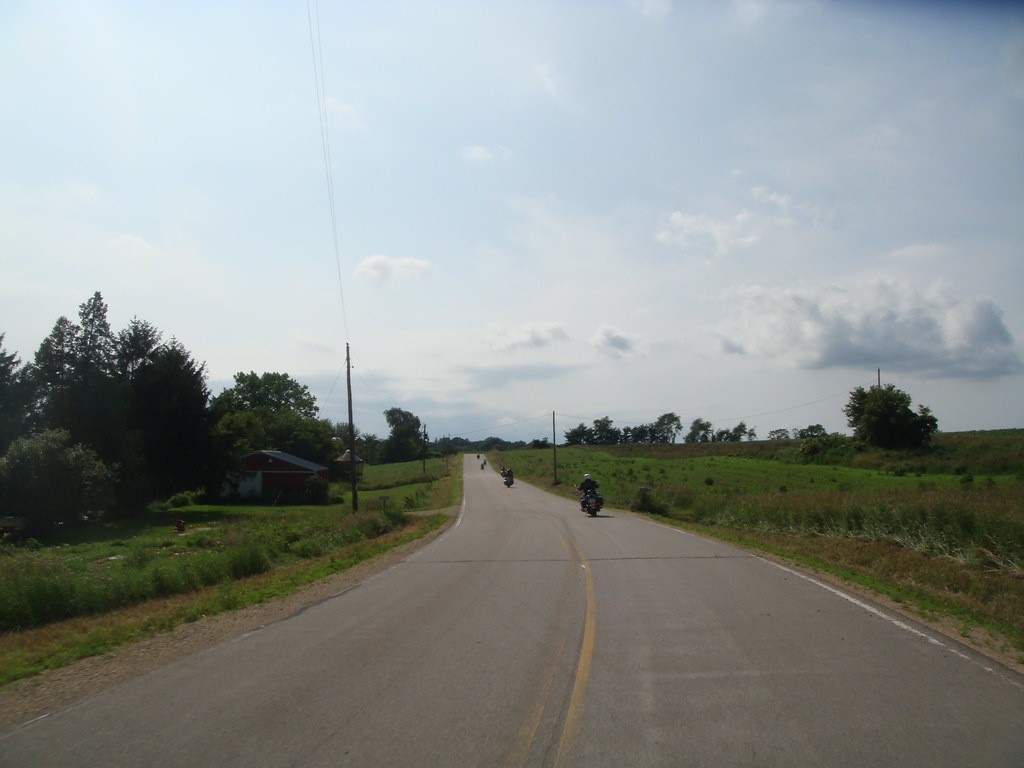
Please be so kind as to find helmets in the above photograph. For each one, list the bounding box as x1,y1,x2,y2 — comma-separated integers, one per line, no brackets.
584,474,591,479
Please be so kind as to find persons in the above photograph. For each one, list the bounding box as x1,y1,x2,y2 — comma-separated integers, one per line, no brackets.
578,473,603,512
484,459,486,465
481,463,484,469
477,455,480,459
501,466,513,480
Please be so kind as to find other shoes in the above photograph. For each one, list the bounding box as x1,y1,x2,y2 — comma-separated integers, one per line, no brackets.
581,508,584,513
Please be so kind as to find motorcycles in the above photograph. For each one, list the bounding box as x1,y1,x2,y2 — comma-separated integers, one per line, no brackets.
574,483,602,516
505,474,513,488
501,469,506,476
